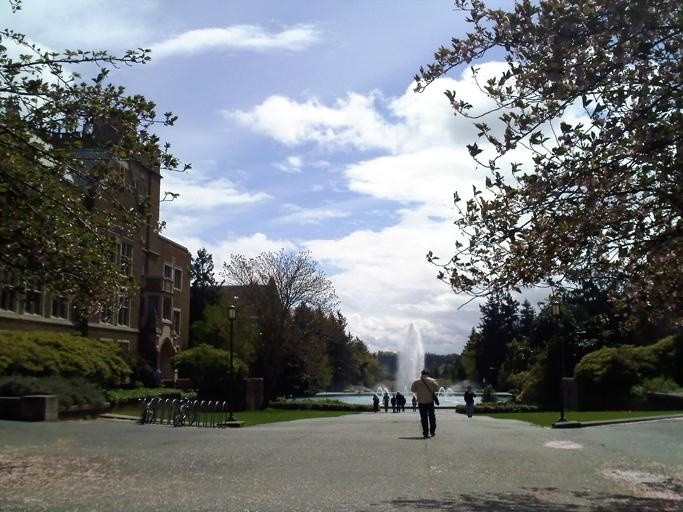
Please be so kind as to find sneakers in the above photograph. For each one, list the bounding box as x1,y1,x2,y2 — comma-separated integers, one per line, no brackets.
424,432,434,437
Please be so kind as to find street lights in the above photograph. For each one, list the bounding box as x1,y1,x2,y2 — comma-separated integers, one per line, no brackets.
551,299,566,422
226,304,238,421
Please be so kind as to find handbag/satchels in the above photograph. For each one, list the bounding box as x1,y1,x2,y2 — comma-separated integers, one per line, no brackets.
432,392,439,405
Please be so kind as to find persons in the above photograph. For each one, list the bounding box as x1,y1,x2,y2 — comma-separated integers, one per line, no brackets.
411,369,440,438
373,391,417,413
464,386,476,417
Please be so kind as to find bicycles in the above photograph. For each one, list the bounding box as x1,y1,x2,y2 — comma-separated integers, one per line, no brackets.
142,395,157,423
174,399,196,428
